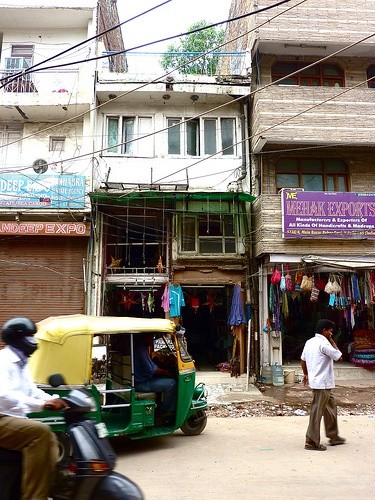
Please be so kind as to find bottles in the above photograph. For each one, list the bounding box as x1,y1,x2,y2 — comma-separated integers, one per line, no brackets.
262,361,284,386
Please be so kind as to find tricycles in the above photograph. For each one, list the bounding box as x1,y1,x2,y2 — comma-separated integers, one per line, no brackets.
23,312,213,470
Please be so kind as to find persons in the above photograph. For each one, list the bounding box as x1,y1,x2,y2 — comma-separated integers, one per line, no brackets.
135,333,176,413
0,316,70,500
301,320,345,450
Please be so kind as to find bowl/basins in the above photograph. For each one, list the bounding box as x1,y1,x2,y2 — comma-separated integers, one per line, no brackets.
294,374,304,382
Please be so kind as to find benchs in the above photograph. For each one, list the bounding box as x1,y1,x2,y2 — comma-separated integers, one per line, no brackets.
108,350,156,428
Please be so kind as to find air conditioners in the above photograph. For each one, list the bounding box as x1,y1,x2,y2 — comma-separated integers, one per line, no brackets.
22,155,54,175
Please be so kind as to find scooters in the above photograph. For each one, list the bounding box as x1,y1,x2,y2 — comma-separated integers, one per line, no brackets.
0,373,146,500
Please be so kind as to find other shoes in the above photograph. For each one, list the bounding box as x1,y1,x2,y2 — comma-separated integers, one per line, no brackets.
305,442,327,450
330,438,346,444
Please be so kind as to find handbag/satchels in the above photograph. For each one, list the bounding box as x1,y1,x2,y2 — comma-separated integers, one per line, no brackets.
280,264,286,292
300,275,313,292
271,263,280,284
295,265,303,284
311,274,319,301
325,273,341,293
316,273,325,290
285,265,292,291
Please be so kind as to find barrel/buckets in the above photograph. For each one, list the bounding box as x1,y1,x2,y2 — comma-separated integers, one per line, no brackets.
284,370,295,384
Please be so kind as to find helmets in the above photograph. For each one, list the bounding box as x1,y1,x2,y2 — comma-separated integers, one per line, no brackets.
2,318,38,354
